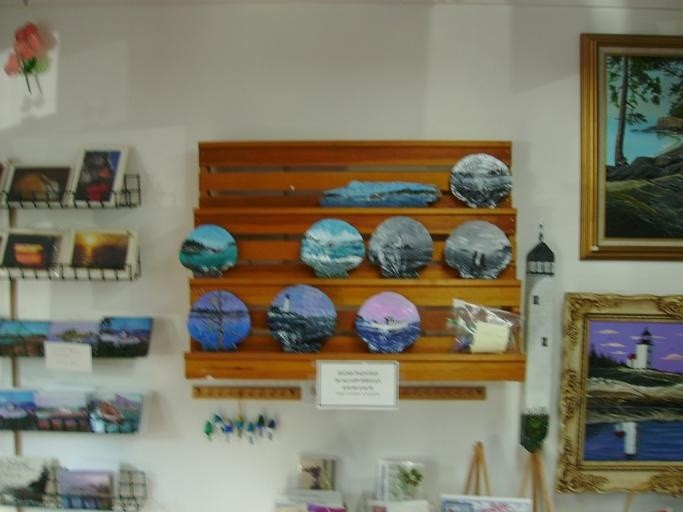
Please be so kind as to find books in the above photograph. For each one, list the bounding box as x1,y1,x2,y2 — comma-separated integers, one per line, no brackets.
274,451,535,512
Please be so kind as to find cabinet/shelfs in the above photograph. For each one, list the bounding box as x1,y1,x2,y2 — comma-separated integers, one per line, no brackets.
0,167,151,512
182,134,528,380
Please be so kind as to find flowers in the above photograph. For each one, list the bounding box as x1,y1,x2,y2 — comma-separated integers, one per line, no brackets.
3,23,46,93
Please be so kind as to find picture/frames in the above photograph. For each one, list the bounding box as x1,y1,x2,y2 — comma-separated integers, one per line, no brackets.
0,141,140,278
315,358,400,411
